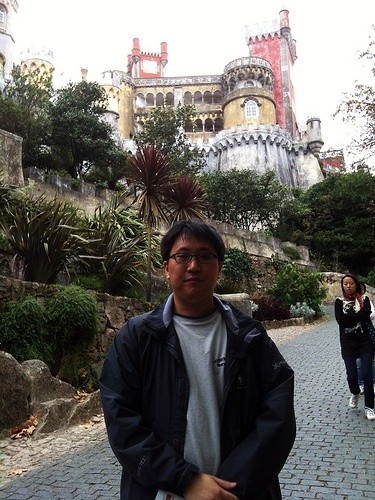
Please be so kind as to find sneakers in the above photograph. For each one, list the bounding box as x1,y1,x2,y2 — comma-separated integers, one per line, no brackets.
349,394,359,408
373,384,375,394
359,384,364,395
364,406,375,420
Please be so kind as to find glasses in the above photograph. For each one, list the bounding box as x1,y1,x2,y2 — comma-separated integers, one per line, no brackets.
168,253,221,264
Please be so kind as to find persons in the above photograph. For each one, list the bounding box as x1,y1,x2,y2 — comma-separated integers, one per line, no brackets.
335,272,374,421
101,219,296,499
